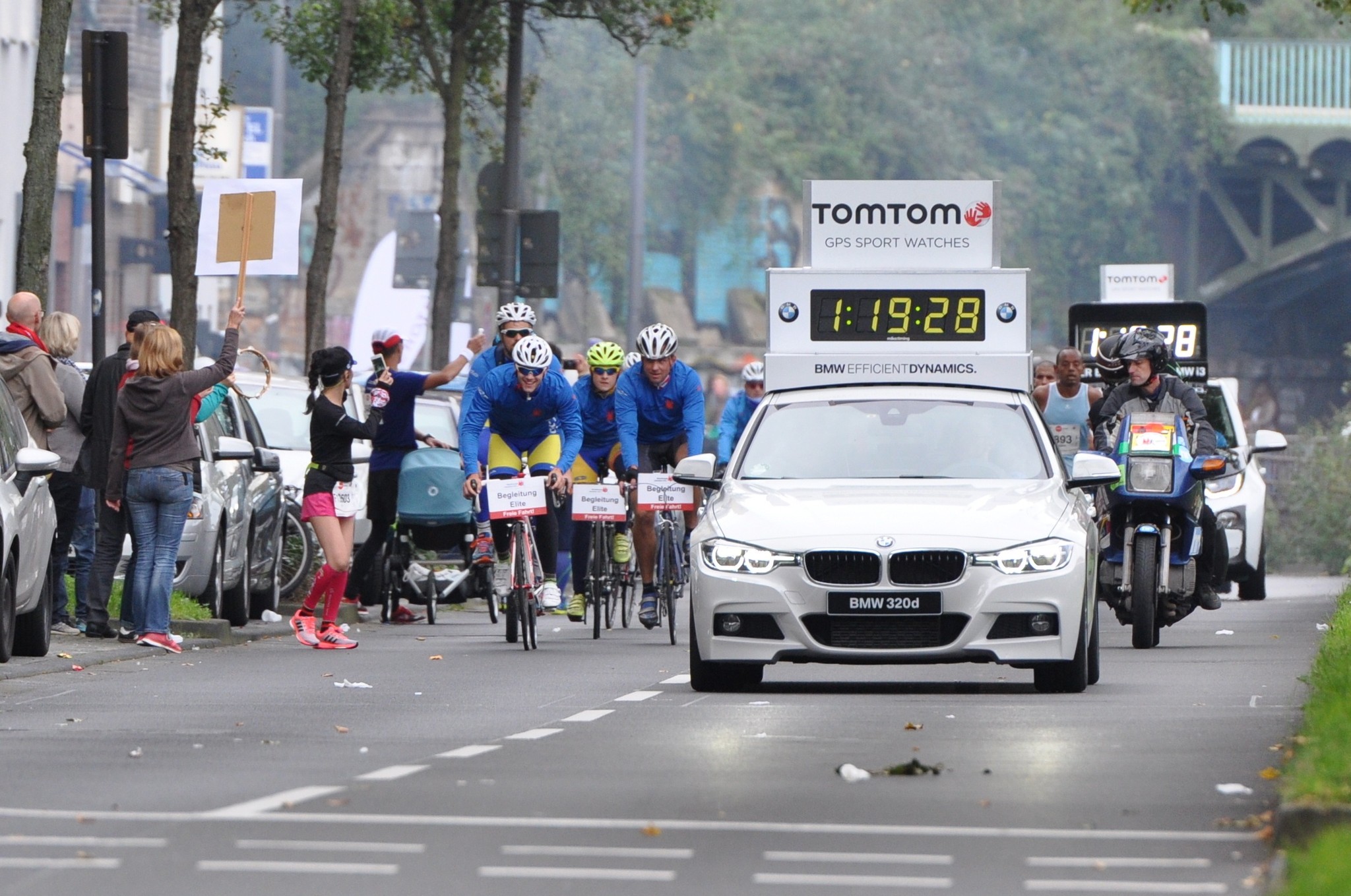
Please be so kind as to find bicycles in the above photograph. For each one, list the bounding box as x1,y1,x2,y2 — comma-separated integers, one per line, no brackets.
469,442,694,648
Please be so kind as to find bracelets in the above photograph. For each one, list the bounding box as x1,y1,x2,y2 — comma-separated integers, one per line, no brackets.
422,433,435,442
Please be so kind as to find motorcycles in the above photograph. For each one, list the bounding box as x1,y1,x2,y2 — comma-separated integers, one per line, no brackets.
1075,410,1227,649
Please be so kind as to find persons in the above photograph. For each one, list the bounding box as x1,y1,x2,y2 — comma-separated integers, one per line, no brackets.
1089,334,1228,590
1033,361,1058,390
1030,346,1104,476
717,359,766,476
453,300,742,628
38,310,86,637
1093,329,1222,610
1242,375,1280,436
168,374,236,642
77,310,159,643
340,328,487,614
117,318,214,642
45,482,99,633
287,346,393,644
0,292,68,452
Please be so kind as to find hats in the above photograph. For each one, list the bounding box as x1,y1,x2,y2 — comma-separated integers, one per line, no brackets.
371,327,404,351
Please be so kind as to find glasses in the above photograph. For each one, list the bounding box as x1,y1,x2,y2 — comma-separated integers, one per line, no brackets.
500,328,533,338
515,363,546,376
589,366,620,375
746,382,763,389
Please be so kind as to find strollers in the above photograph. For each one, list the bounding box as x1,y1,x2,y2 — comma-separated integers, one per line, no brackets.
379,443,500,626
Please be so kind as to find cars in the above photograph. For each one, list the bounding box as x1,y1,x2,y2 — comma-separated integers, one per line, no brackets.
66,355,458,626
671,380,1124,693
1181,376,1289,601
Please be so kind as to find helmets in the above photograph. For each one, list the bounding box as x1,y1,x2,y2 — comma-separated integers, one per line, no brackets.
1095,328,1169,385
635,322,678,360
511,335,552,368
741,361,764,382
622,351,641,371
495,302,537,327
587,341,625,366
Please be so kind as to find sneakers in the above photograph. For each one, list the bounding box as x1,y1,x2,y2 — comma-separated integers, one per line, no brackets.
682,532,692,564
542,581,561,608
47,615,182,654
469,532,497,564
638,588,659,625
611,533,632,563
289,609,321,646
1193,579,1222,613
380,606,428,625
312,624,358,650
498,602,507,612
552,602,567,615
567,594,586,619
340,593,369,615
493,558,512,597
